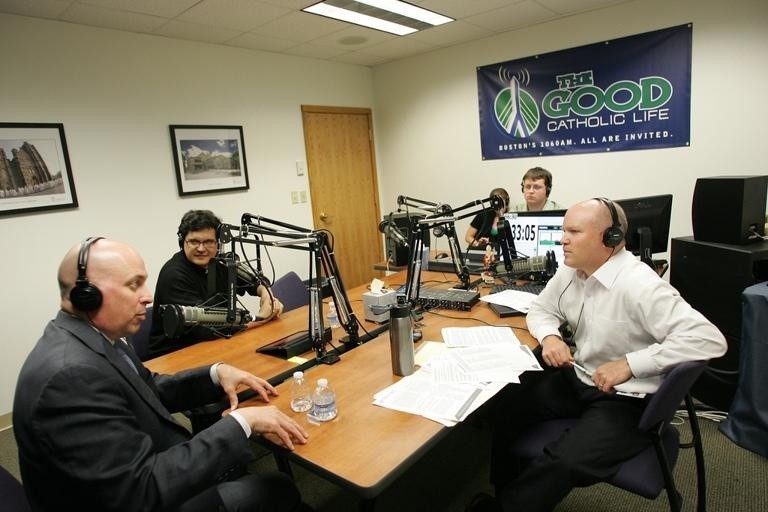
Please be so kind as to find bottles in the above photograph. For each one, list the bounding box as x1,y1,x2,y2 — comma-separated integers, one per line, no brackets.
327,302,343,328
289,368,313,415
309,378,338,425
388,305,418,378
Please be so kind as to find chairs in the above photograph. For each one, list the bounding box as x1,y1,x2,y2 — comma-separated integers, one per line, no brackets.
271,273,309,313
605,360,709,511
125,305,154,361
0,466,25,511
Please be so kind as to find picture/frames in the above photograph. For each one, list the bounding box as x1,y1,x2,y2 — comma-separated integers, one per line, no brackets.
170,124,249,196
1,122,78,216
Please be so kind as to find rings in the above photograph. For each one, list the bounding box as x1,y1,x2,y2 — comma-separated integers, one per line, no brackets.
598,383,604,388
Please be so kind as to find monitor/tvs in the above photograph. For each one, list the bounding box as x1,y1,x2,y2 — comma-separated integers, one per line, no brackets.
494,209,567,262
612,194,673,278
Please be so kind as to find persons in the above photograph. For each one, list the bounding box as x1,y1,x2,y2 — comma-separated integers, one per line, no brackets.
398,198,730,512
11,236,311,510
465,186,510,259
509,167,566,213
145,210,283,353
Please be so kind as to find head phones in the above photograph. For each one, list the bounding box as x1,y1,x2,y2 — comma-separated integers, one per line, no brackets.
592,197,623,248
70,237,105,310
177,232,184,249
521,167,552,198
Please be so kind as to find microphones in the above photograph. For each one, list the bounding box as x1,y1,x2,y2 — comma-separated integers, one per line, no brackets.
466,223,487,261
378,220,407,247
159,304,256,340
492,196,504,210
489,251,557,277
219,251,270,286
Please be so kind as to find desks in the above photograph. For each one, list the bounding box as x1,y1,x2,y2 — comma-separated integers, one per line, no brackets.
222,280,539,512
143,270,482,416
374,249,456,274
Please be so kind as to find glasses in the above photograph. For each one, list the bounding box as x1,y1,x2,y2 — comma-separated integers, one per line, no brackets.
183,240,218,248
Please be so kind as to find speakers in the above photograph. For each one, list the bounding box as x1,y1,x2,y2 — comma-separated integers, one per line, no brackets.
669,236,768,411
691,175,768,246
384,212,431,266
